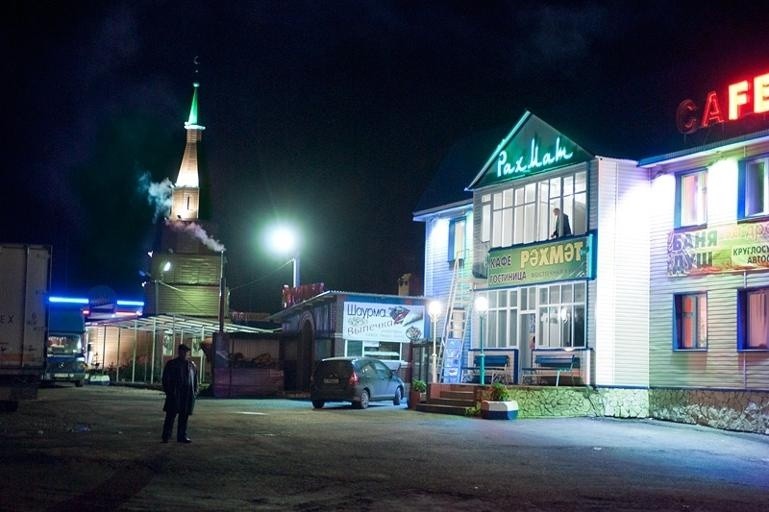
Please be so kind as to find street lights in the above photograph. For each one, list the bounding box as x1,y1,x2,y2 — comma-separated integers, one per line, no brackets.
428,298,442,384
475,294,490,385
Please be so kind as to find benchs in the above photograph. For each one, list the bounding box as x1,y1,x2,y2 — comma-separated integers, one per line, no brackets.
521,354,578,388
461,354,510,384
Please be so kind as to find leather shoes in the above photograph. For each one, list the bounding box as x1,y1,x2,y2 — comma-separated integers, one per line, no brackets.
161,439,170,443
177,436,192,442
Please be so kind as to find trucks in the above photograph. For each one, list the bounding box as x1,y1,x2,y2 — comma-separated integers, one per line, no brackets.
43,302,90,389
0,243,53,411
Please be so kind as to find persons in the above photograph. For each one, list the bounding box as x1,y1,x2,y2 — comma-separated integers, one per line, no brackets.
158,346,199,443
551,208,571,239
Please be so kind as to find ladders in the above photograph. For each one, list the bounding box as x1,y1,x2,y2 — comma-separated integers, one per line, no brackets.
437,249,474,383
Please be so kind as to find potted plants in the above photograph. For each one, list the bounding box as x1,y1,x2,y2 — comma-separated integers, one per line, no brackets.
480,383,519,420
409,381,428,410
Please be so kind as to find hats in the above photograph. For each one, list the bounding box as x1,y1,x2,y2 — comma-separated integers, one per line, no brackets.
178,344,190,351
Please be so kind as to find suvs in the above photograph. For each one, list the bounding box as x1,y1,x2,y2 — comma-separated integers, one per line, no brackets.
308,356,405,410
